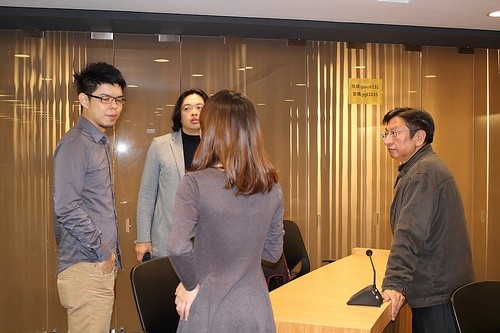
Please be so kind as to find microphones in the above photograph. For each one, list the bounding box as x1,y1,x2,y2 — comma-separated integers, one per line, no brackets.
347,250,383,307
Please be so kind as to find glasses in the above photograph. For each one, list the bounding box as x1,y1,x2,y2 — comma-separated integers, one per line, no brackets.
381,129,413,140
86,94,126,105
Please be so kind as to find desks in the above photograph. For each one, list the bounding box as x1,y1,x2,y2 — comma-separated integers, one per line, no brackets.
270,248,412,333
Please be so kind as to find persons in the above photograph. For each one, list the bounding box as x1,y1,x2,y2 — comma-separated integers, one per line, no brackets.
167,89,285,332
54,61,126,333
135,88,208,264
382,107,475,333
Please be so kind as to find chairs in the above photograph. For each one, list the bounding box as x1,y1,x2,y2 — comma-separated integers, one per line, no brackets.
261,220,311,292
130,257,180,333
449,280,500,333
